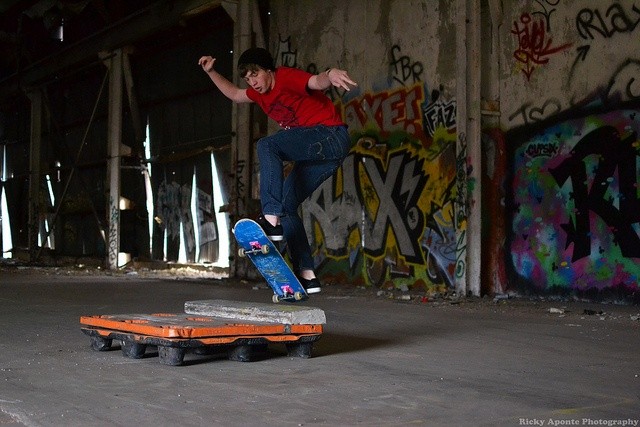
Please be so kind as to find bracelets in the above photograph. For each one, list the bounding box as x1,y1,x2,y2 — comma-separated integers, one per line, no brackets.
326,68,332,77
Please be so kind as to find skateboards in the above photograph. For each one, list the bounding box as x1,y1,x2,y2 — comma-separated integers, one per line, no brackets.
234,218,309,303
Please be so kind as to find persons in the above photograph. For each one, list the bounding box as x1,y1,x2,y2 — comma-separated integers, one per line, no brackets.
198,48,359,295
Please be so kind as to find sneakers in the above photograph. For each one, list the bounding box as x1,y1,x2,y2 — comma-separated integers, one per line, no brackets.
232,215,283,242
274,277,321,296
